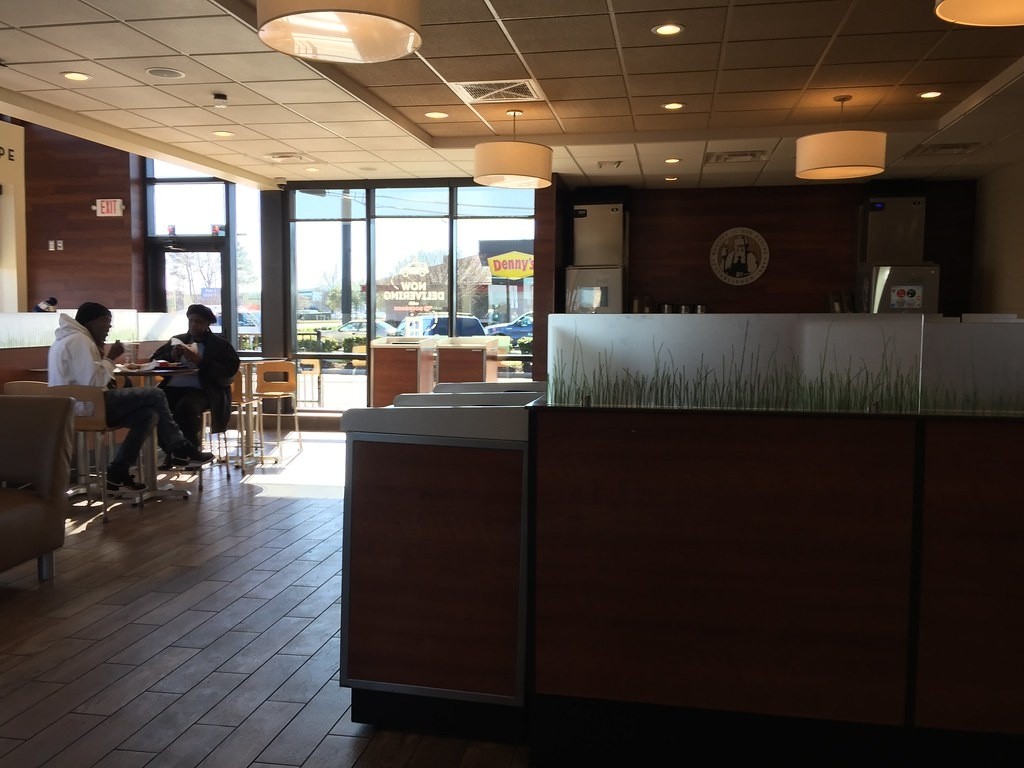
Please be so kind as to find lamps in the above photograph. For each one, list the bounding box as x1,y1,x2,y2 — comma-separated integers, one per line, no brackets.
255,0,422,64
471,110,553,188
795,95,887,180
934,0,1024,26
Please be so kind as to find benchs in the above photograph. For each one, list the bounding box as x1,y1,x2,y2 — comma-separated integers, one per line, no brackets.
0,395,76,583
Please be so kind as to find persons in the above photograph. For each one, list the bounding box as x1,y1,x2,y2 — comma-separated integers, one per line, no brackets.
148,304,241,471
47,302,215,494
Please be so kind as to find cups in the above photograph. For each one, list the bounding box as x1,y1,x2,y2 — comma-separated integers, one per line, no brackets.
124,343,139,363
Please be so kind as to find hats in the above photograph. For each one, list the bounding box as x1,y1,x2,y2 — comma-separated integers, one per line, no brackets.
34,297,59,313
74,302,112,325
186,304,217,324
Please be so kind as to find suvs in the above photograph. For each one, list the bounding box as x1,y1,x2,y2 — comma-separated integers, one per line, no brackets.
394,311,487,338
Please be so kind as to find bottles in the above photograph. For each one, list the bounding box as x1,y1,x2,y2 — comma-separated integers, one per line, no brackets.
632,297,705,313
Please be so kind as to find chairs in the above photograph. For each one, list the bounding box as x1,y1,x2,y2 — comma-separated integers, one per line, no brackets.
4,360,300,525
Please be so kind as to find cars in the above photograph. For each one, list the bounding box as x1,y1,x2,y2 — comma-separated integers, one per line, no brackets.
316,319,397,340
484,311,534,347
207,305,331,349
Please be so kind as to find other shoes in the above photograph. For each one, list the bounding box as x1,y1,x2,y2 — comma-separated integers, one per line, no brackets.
157,455,172,471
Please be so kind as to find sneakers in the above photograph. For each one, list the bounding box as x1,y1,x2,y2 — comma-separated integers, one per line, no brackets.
171,441,215,468
106,474,148,495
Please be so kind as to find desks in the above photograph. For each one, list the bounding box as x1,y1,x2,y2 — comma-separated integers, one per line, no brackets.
27,368,199,505
